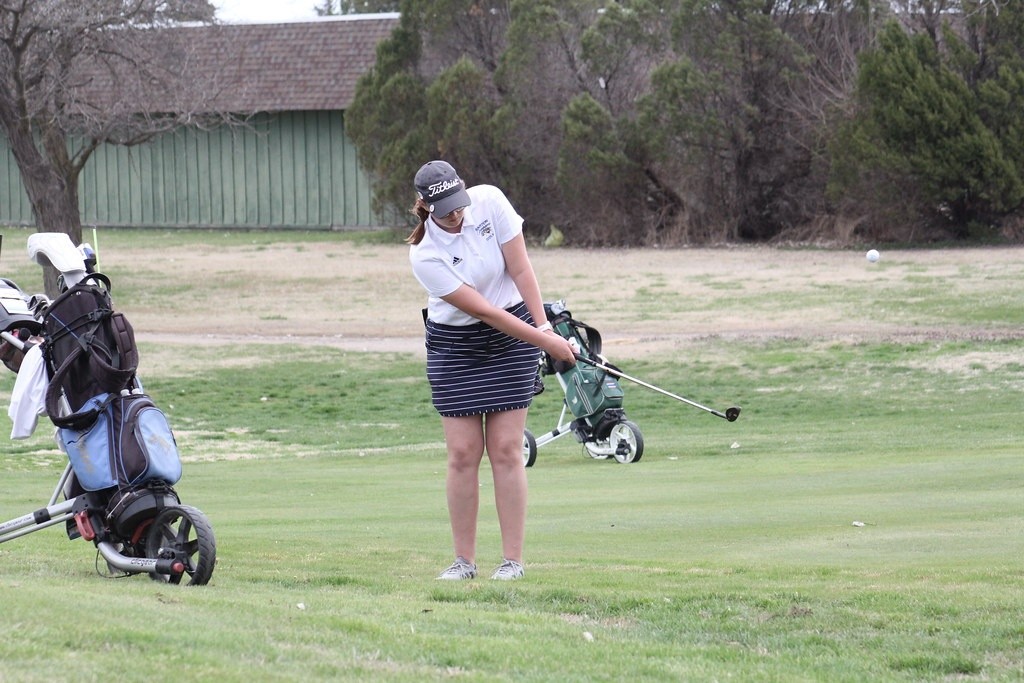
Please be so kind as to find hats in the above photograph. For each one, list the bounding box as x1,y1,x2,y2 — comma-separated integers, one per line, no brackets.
413,160,470,219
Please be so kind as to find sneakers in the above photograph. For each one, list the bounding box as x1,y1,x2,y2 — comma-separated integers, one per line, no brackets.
436,556,478,581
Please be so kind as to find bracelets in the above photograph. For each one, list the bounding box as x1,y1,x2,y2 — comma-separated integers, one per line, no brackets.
536,320,555,334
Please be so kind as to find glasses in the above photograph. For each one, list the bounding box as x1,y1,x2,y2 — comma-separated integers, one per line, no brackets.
441,206,465,217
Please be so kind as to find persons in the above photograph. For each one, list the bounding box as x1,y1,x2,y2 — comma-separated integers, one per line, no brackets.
401,159,582,581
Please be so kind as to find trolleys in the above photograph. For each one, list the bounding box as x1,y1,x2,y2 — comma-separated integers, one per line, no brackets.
524,301,644,465
0,229,215,586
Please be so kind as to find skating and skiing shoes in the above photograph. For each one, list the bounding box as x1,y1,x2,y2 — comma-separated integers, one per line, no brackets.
488,560,525,580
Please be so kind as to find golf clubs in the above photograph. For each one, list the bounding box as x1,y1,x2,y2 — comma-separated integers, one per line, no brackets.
571,352,742,424
